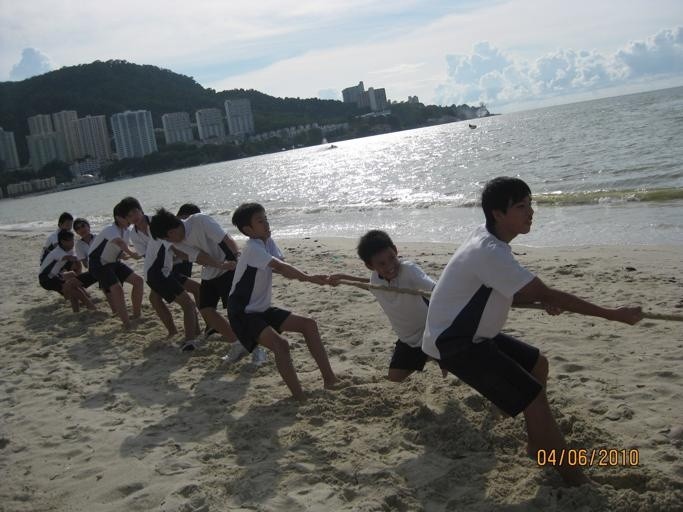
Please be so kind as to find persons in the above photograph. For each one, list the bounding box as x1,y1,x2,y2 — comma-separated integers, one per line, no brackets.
62,217,123,318
37,228,91,313
118,195,194,343
39,211,83,301
225,200,346,402
328,228,447,384
147,204,267,367
419,174,641,463
86,201,146,329
141,201,202,349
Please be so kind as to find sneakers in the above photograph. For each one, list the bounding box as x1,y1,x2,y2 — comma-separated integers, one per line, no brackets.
181,340,197,352
224,341,250,364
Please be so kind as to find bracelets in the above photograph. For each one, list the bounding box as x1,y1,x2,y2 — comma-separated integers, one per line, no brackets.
220,260,226,268
233,252,241,259
